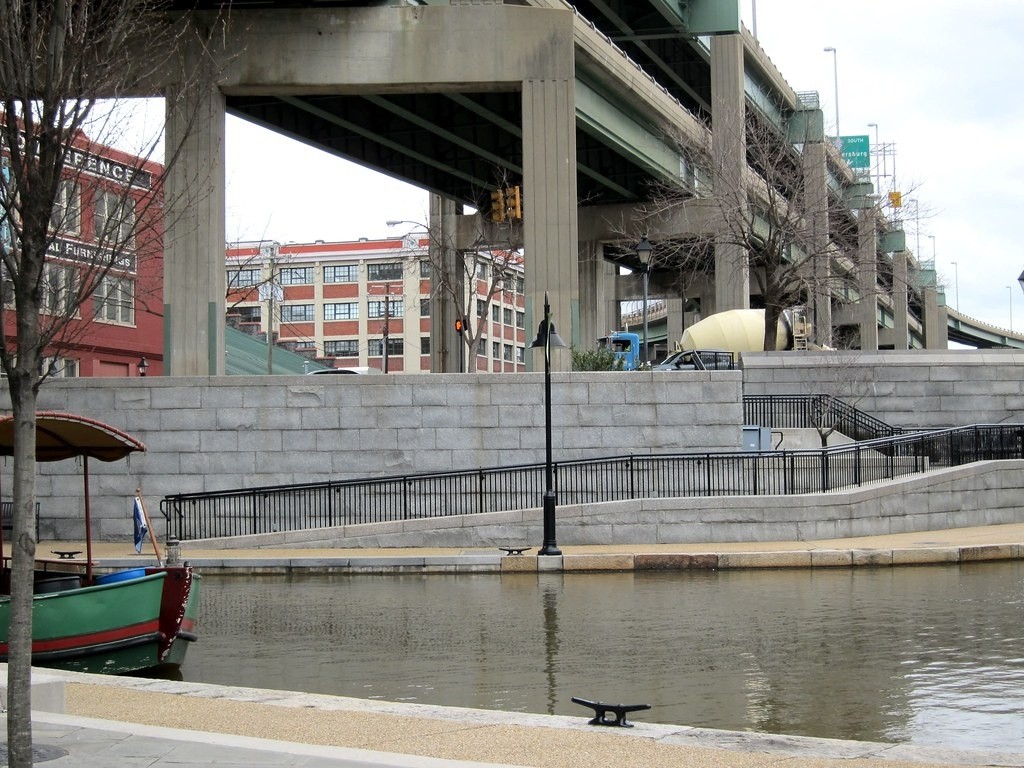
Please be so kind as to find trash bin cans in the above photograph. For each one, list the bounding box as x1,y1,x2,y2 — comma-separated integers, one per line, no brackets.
596,331,640,371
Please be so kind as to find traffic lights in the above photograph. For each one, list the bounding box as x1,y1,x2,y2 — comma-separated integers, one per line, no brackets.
491,190,505,223
507,184,522,221
455,318,462,332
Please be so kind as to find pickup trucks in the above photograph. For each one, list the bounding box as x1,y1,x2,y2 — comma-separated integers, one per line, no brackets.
647,348,739,371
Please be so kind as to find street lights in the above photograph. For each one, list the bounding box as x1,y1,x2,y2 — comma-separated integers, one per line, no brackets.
867,123,880,208
908,199,919,263
928,235,936,270
824,47,840,153
527,291,569,555
951,262,959,311
1006,286,1012,331
636,231,652,371
385,219,447,373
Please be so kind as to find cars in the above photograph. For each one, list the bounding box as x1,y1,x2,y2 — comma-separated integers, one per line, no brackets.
308,370,360,374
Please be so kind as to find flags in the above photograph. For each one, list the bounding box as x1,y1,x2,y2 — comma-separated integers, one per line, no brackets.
132,496,148,555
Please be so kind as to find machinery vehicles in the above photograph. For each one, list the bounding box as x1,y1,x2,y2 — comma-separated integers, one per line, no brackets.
594,303,838,371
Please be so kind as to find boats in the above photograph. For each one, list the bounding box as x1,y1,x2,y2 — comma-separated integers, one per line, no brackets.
1,410,202,675
